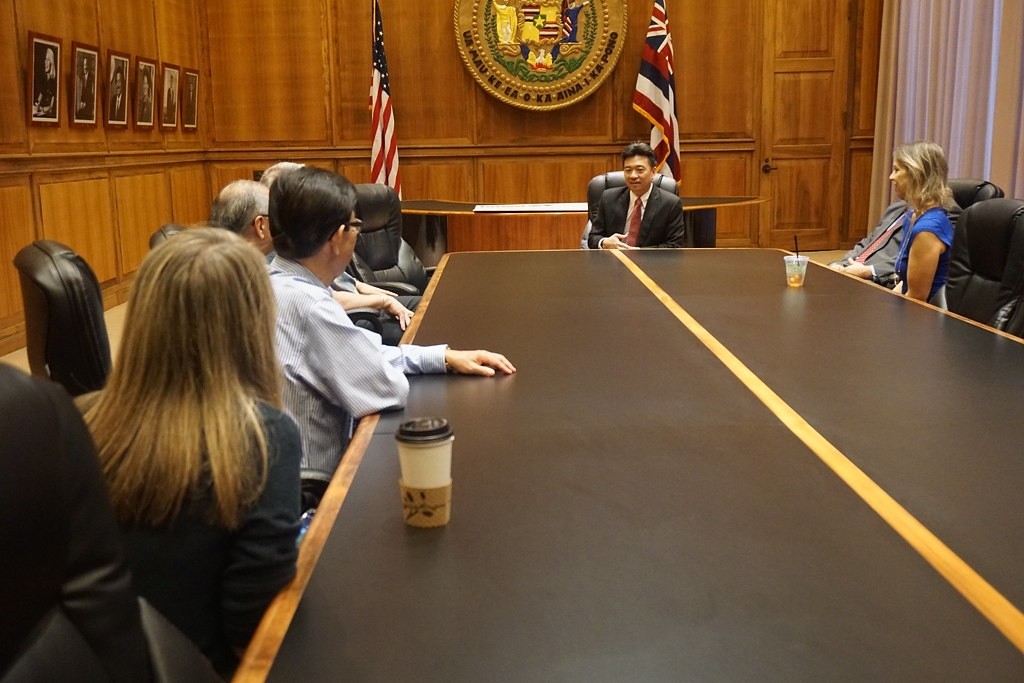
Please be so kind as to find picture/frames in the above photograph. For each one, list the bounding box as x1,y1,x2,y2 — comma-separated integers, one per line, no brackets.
135,55,156,132
71,40,99,129
28,28,62,128
160,61,180,132
107,48,131,131
181,66,200,133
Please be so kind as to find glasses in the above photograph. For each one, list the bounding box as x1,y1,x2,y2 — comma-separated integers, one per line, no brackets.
328,218,364,241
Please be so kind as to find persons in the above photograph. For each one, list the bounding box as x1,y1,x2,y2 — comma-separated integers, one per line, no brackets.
587,143,684,249
34,48,56,118
167,75,175,124
139,68,152,122
186,82,195,125
207,161,516,517
826,141,964,311
72,226,303,670
76,57,93,120
110,66,125,122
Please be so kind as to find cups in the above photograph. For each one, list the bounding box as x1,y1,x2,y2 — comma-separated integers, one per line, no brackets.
394,415,456,488
784,255,809,287
398,478,453,527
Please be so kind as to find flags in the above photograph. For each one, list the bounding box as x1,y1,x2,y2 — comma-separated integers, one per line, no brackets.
370,0,401,201
633,0,682,188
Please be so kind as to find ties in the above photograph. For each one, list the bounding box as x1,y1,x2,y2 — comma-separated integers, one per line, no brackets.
854,215,902,263
628,198,642,246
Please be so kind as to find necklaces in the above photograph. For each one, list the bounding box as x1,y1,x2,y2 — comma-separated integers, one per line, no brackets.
894,213,917,286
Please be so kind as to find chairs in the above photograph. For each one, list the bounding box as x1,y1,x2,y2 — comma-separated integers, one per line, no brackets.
13,239,111,397
347,182,438,297
945,195,1024,332
946,179,1004,213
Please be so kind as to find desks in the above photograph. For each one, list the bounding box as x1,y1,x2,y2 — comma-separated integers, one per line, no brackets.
230,247,1024,683
